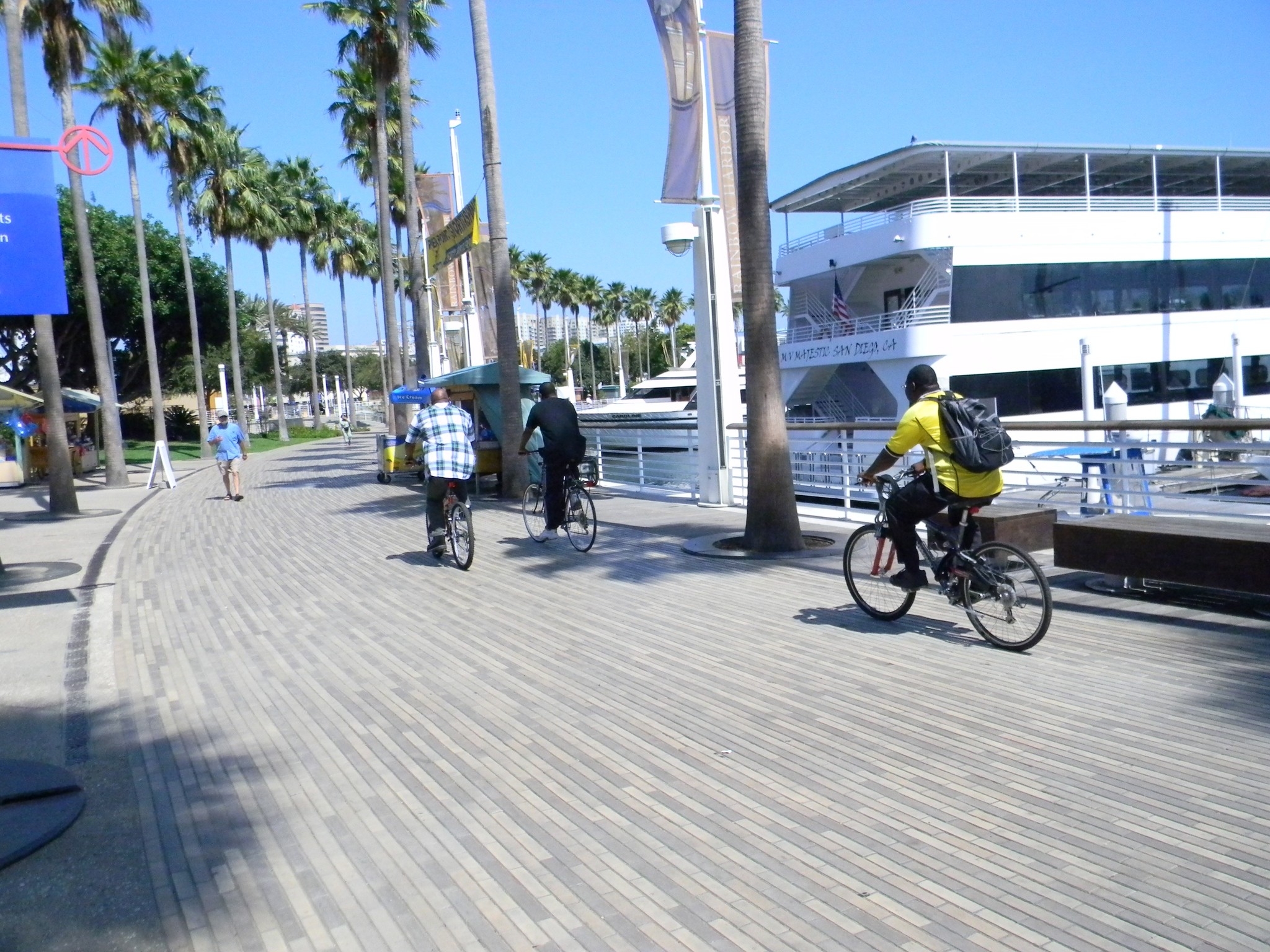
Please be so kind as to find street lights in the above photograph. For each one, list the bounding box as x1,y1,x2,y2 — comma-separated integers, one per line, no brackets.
660,203,742,503
443,310,486,371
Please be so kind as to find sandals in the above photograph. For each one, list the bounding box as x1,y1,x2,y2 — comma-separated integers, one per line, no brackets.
235,494,244,501
224,493,233,500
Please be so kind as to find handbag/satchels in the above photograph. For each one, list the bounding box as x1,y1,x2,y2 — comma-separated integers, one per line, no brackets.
344,427,349,431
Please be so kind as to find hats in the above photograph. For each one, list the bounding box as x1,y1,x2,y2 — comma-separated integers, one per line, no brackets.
217,410,228,417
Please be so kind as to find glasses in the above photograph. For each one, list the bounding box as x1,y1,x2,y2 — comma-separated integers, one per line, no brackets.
901,383,909,390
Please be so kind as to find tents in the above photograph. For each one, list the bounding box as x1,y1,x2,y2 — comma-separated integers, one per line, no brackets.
19,387,124,477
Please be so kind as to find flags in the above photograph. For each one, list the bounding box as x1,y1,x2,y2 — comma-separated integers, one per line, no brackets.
832,275,852,327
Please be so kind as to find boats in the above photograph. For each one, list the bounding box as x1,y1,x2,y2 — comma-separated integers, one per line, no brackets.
575,329,793,452
767,141,1270,503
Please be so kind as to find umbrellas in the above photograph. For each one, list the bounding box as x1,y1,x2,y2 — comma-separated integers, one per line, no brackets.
389,381,436,407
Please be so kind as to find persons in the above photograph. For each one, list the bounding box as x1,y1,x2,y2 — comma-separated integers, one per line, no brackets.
405,388,475,553
340,414,352,447
207,410,247,501
857,364,1015,596
518,382,586,541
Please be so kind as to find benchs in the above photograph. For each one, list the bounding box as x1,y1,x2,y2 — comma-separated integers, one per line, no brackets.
1052,513,1270,598
927,504,1057,556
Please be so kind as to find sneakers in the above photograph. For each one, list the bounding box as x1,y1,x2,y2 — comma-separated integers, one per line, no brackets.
539,526,559,540
890,568,929,589
570,493,582,511
459,507,473,521
427,535,447,553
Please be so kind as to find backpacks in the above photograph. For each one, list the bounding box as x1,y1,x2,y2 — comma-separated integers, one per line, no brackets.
917,391,1015,473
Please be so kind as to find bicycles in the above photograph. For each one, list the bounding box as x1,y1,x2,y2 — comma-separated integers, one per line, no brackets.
839,471,1054,652
406,456,474,572
514,445,601,554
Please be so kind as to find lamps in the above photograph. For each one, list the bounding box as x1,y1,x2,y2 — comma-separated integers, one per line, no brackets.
773,270,782,276
895,235,905,243
